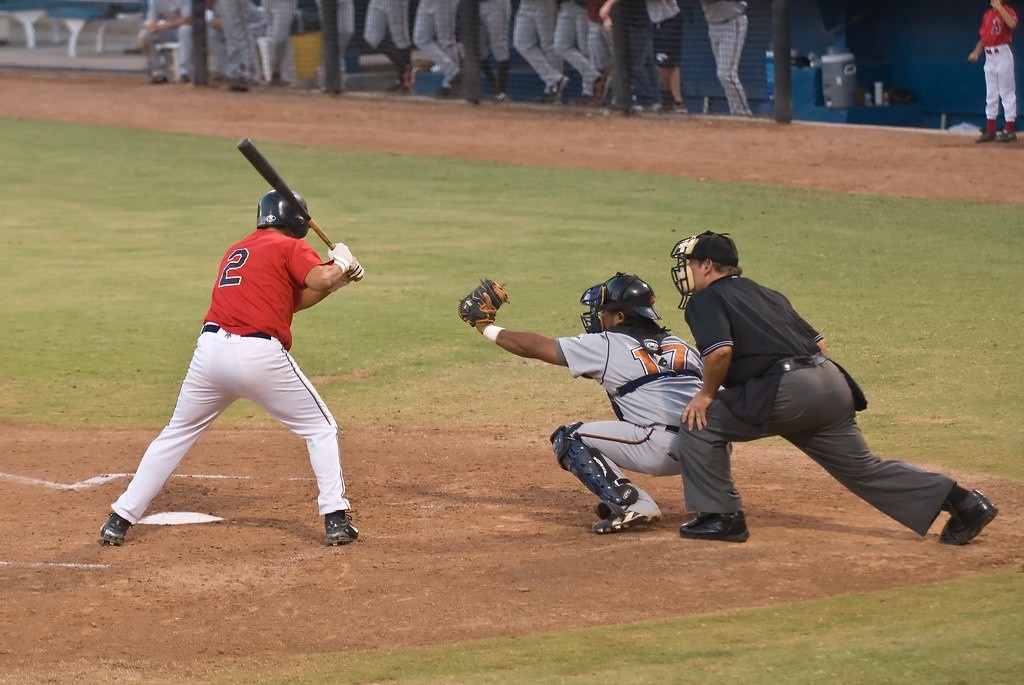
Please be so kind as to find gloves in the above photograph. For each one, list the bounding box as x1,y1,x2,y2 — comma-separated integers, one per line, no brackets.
328,242,352,273
340,257,364,282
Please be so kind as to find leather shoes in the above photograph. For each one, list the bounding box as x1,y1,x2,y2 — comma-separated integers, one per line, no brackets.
939,490,997,544
681,512,749,542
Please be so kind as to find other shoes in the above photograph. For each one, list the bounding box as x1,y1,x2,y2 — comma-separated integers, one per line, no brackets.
977,133,996,142
651,94,689,113
399,61,410,88
591,77,612,104
437,76,465,98
999,133,1016,142
546,77,569,104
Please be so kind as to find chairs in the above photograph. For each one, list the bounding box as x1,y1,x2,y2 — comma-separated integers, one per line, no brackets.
0,0,321,96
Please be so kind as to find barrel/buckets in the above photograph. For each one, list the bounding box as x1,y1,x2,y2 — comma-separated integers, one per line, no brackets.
820,53,857,109
288,33,322,90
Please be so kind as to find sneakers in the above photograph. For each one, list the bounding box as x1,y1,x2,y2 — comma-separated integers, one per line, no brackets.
591,506,660,534
99,512,131,545
325,508,358,546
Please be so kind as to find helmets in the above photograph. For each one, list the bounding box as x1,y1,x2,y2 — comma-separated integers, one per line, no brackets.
601,275,663,320
257,189,309,237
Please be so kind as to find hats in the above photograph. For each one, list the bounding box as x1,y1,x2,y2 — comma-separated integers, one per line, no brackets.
684,232,739,266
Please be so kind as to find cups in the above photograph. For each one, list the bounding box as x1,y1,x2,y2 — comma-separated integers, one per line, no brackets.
874,82,882,105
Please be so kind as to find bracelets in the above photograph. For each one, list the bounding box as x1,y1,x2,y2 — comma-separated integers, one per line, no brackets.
483,325,506,343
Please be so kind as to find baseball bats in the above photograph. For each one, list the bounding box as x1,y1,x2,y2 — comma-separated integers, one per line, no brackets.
236,136,364,282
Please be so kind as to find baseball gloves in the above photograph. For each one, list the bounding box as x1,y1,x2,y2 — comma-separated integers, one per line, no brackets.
456,277,510,328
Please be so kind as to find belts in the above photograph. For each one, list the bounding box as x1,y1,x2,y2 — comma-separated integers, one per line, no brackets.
203,325,271,341
666,425,679,433
778,353,827,373
987,49,999,53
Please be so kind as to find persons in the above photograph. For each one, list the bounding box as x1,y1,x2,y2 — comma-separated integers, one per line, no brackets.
669,230,998,546
102,186,364,548
138,0,300,93
965,0,1019,144
316,0,756,116
457,270,732,534
